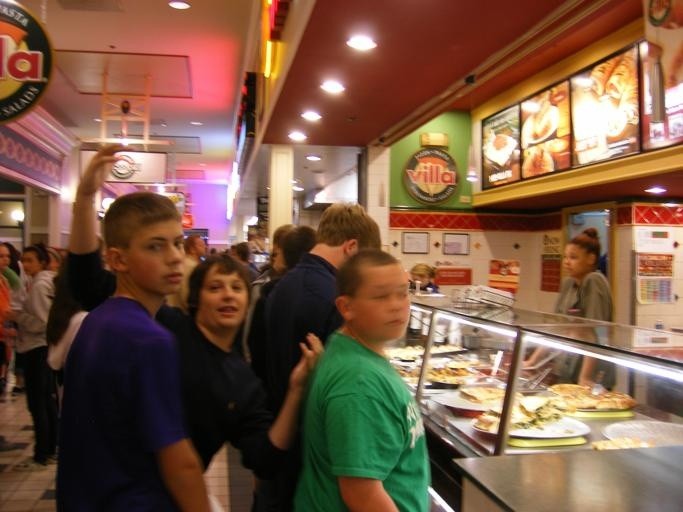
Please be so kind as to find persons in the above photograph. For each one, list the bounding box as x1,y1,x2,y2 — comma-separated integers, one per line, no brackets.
244,200,381,512
51,139,322,481
409,263,438,293
56,190,212,512
517,226,612,389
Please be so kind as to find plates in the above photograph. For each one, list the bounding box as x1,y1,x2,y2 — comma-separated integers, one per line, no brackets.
520,107,564,144
604,419,680,443
483,134,519,165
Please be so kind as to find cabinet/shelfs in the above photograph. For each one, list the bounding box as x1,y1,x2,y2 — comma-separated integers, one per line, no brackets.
380,287,682,511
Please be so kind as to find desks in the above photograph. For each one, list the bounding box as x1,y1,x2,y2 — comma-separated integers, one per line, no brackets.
449,436,682,512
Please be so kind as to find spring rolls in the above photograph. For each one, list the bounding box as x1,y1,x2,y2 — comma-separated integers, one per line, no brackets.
589,53,639,120
